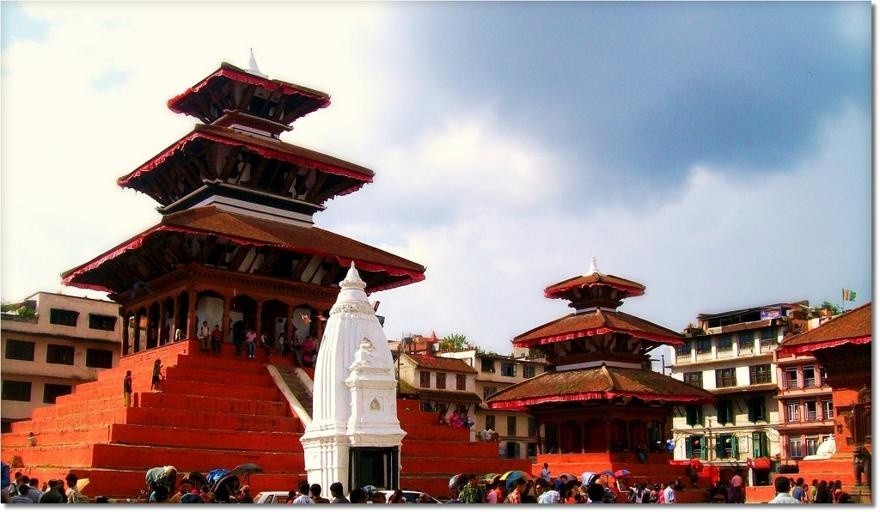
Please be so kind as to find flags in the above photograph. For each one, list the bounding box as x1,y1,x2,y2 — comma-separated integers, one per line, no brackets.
843,288,857,301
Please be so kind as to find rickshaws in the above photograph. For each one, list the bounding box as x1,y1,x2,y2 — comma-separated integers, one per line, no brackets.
554,472,579,492
478,472,501,496
581,472,600,491
180,471,210,494
145,464,177,501
602,470,635,502
448,472,476,500
207,463,262,503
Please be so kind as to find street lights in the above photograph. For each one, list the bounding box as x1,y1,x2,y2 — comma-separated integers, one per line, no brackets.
704,428,714,460
396,343,403,398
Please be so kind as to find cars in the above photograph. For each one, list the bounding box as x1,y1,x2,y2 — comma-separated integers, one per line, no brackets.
250,489,301,505
343,488,444,505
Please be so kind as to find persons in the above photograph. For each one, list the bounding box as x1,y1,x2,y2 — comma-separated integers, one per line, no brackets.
175,320,318,367
438,409,498,441
124,371,132,408
151,360,164,391
